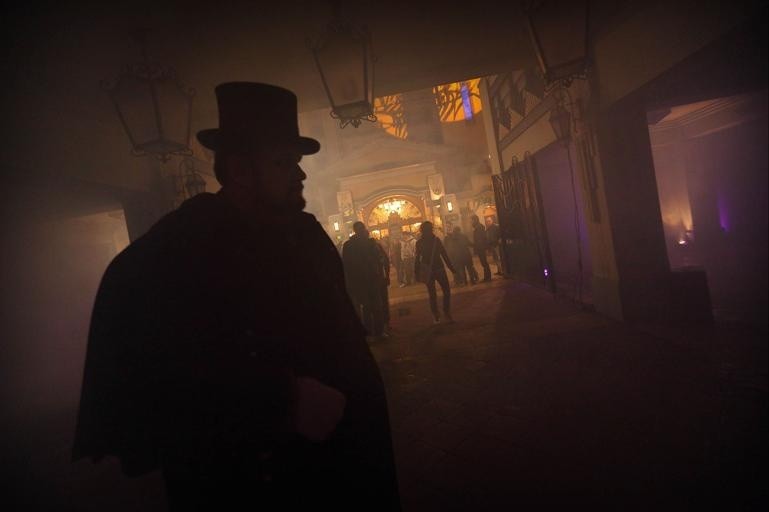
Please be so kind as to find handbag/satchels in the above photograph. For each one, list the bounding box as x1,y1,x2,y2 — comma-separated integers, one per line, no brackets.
417,236,436,283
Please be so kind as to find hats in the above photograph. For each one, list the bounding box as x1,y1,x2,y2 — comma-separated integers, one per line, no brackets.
195,81,321,157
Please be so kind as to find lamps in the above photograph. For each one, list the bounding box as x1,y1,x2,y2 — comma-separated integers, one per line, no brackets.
548,86,578,149
169,159,207,215
301,0,378,131
98,26,198,164
678,229,693,245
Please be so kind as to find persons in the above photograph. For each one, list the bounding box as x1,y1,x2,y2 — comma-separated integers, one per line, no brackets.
71,80,402,511
337,216,501,341
